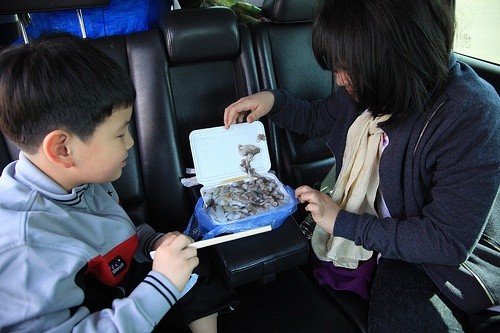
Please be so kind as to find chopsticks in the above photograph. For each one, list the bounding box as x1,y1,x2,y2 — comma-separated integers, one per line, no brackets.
150,225,272,259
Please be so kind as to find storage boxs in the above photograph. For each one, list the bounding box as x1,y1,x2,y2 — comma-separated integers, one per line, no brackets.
189,120,295,226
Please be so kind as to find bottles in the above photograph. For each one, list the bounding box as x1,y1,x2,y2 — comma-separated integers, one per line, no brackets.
299,186,334,239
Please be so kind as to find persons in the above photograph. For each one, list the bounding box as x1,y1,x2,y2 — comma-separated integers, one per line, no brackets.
0,29,200,333
178,1,500,333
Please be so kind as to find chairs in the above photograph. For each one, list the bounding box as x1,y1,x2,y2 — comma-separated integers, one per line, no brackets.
0,0,378,333
